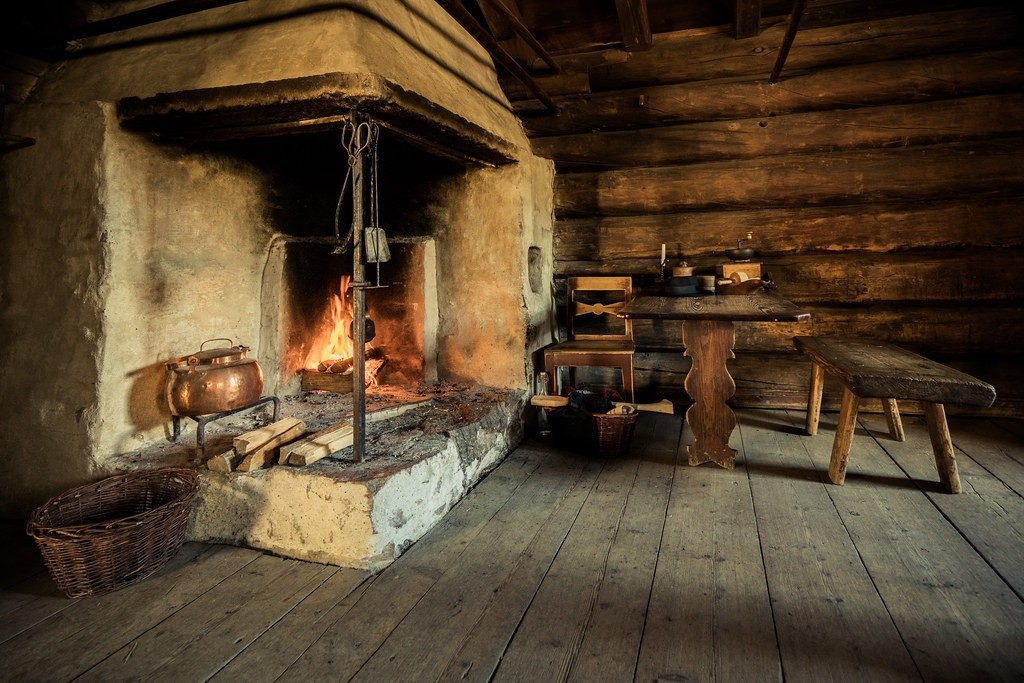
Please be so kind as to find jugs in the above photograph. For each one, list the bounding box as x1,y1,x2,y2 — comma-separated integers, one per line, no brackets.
668,266,703,297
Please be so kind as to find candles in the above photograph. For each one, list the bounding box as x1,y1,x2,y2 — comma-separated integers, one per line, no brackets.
661,242,665,268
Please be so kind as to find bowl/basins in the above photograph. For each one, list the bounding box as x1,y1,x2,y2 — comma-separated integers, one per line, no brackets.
725,248,753,258
715,278,770,294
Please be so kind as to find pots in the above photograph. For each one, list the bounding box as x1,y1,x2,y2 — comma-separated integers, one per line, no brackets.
165,338,263,416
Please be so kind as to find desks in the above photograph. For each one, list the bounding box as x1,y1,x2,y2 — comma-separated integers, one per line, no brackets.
616,280,811,469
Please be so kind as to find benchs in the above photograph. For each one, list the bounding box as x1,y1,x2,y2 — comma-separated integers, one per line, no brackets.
792,335,996,494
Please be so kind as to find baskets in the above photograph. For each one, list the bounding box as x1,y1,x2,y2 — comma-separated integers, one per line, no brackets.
580,409,639,460
26,467,200,599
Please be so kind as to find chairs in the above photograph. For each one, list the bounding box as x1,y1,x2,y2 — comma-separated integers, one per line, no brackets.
544,276,632,403
